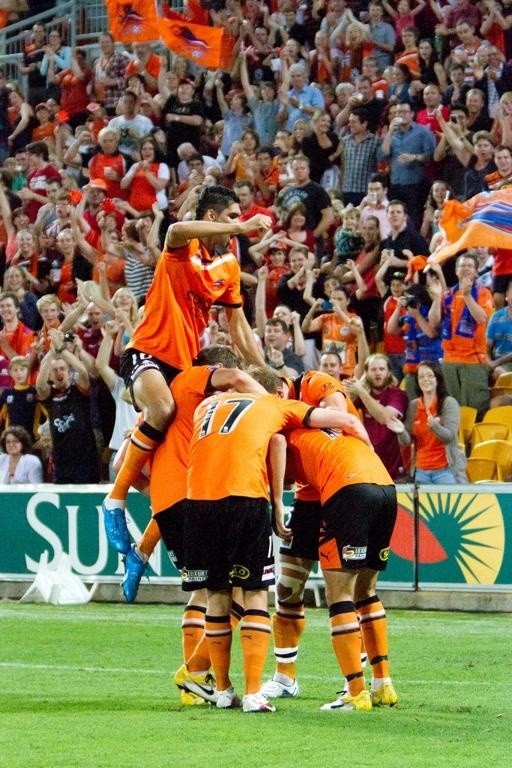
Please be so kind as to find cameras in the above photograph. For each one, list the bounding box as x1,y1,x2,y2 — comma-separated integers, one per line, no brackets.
62,333,74,342
320,302,333,311
405,296,416,309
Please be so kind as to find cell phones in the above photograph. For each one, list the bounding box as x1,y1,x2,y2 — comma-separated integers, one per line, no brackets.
449,114,458,124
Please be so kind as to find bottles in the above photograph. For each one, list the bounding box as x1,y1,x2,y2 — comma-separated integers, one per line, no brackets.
51,260,61,282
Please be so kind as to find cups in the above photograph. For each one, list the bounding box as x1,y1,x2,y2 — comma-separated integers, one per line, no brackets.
368,193,377,210
104,167,111,175
394,118,402,126
353,93,364,106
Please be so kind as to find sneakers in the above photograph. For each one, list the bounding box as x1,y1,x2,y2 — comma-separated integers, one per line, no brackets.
178,664,275,712
260,677,299,698
120,543,151,602
320,685,372,712
370,682,397,708
101,497,131,554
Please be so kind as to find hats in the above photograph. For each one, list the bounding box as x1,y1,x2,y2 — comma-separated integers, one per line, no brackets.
267,241,286,254
81,178,108,194
479,373,512,394
177,78,196,92
35,103,50,112
387,271,407,283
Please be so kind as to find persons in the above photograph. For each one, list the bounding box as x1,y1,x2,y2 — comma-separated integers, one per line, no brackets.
1,1,512,488
103,183,277,606
150,341,273,715
176,363,377,717
248,361,370,701
262,418,407,713
